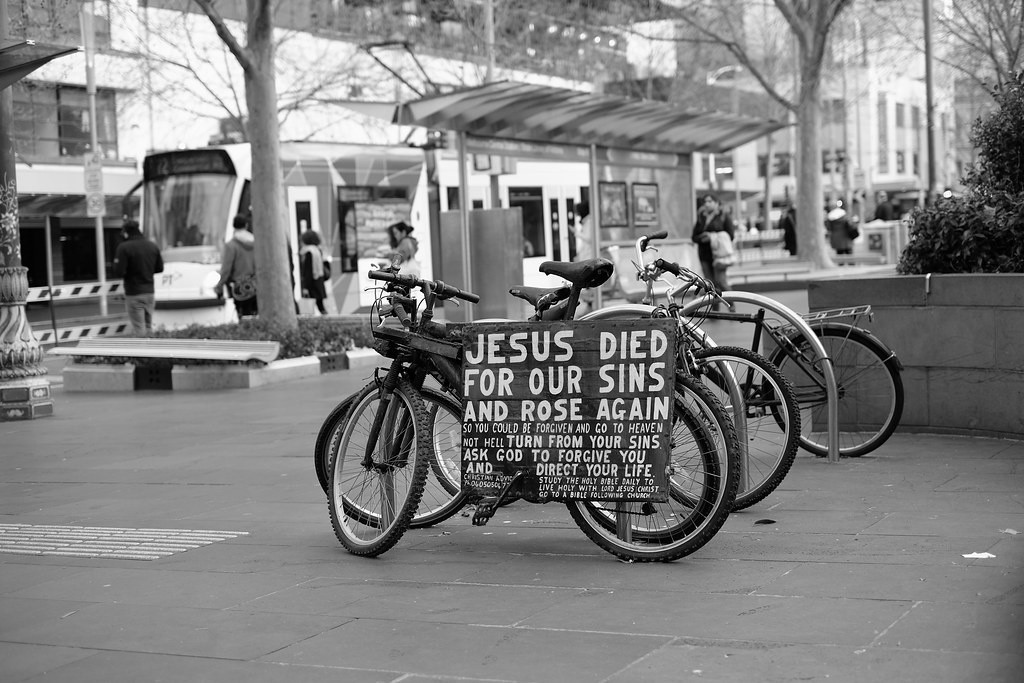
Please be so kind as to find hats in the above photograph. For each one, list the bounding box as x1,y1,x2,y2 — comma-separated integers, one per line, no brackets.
122,220,139,232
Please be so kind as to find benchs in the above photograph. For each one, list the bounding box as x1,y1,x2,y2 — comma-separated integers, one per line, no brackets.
47,334,281,364
609,241,811,297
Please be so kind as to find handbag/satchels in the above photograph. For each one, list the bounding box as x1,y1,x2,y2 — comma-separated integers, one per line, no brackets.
846,222,859,239
298,294,316,314
324,261,331,280
230,275,256,302
397,259,421,279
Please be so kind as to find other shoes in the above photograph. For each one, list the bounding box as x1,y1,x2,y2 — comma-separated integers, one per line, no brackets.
576,307,592,319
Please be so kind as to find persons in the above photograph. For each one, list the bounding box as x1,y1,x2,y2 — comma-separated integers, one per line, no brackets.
692,194,734,320
215,213,258,317
825,198,854,266
114,221,163,331
301,229,326,313
569,198,594,262
874,191,904,221
779,202,798,254
389,222,418,298
